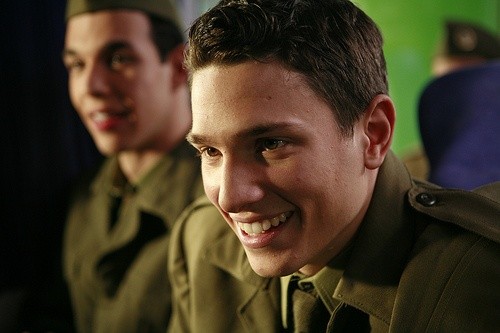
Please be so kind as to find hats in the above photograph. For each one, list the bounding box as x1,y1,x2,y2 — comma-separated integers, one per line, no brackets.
66,0,184,38
442,20,500,60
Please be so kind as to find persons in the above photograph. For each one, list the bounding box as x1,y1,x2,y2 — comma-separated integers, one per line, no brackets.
63,0,207,333
166,0,500,333
